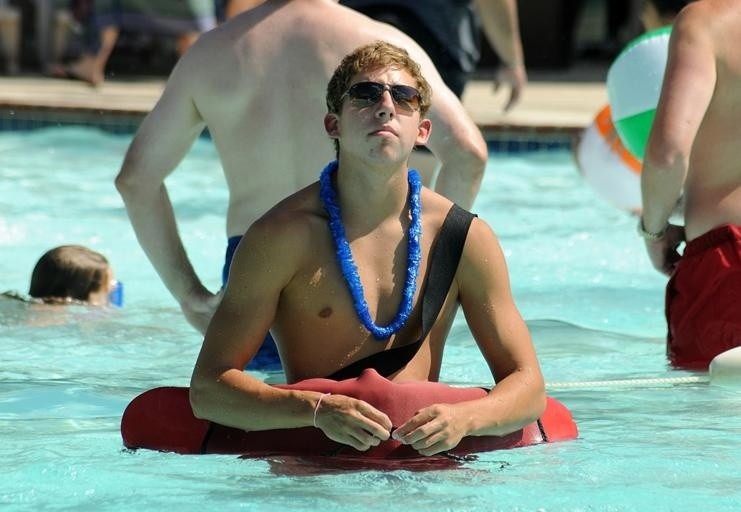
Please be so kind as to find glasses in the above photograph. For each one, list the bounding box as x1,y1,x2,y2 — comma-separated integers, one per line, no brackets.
339,82,423,112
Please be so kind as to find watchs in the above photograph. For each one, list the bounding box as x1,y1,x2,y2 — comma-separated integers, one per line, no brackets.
636,218,670,243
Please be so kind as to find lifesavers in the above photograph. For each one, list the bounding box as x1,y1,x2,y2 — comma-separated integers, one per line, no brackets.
121,368,578,456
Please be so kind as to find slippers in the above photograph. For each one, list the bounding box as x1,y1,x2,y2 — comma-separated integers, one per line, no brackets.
39,60,101,87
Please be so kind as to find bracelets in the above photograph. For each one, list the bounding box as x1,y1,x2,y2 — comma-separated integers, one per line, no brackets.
312,392,332,428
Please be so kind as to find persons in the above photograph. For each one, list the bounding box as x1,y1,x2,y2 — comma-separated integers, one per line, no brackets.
636,1,739,374
571,0,685,219
2,1,254,87
1,244,124,332
339,0,527,188
188,41,548,479
115,0,489,369
